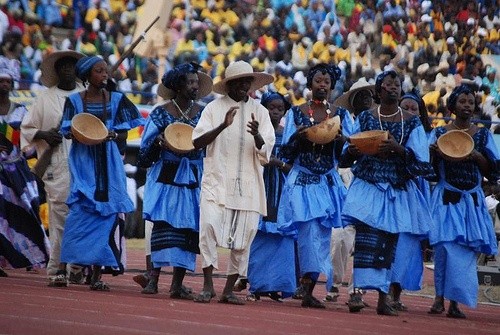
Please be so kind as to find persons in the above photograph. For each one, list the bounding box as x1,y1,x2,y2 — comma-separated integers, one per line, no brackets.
0,0,500,133
243,92,296,302
0,68,52,278
192,61,275,304
426,86,500,318
57,56,145,292
344,66,430,317
20,49,86,287
133,63,214,299
279,63,353,310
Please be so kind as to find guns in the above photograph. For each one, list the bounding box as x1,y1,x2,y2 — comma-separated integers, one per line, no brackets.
31,16,160,180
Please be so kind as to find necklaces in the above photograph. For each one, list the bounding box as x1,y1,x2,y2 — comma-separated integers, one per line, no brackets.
171,98,194,121
377,103,403,152
452,121,473,131
82,88,109,202
307,98,331,162
6,99,14,120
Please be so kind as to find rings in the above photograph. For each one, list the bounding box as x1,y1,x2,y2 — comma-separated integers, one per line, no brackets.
109,137,112,140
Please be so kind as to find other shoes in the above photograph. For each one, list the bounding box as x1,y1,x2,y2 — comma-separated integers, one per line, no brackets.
446,306,467,319
25,266,39,274
292,287,305,300
70,271,83,282
386,294,408,312
431,301,445,313
90,281,112,291
197,288,214,303
233,280,246,292
376,302,399,316
55,270,68,286
0,268,8,277
323,282,342,302
347,291,365,313
220,294,245,305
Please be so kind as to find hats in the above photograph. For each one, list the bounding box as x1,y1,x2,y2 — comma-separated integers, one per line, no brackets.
213,60,274,95
42,49,88,83
157,71,213,100
334,78,375,108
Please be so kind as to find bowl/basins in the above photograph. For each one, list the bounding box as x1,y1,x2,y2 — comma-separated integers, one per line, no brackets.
71,112,108,146
437,130,474,161
163,122,196,154
350,130,389,155
302,115,341,145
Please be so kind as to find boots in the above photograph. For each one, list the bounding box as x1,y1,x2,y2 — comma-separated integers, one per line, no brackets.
141,255,161,294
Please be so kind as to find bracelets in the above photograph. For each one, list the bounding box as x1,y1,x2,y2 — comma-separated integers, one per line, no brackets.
113,132,118,140
278,161,285,170
348,148,352,154
